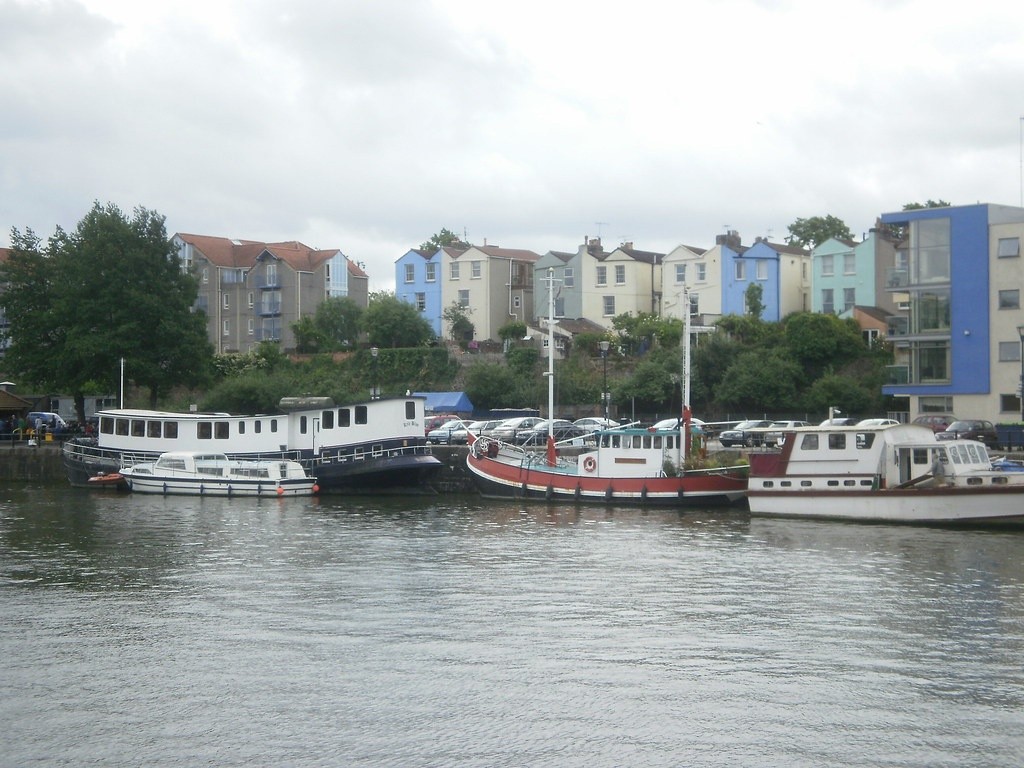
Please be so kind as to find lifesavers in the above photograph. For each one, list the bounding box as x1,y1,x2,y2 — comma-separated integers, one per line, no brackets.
584,456,596,472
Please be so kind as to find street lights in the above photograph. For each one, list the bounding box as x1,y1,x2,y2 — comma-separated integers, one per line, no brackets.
370,346,383,400
598,341,612,430
1015,326,1024,420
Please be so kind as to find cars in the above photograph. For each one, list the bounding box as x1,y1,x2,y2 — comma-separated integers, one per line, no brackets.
910,415,959,432
425,415,461,438
490,416,545,442
763,421,813,448
647,417,714,438
451,429,468,441
718,420,775,447
427,419,476,444
854,418,901,428
816,417,861,427
933,420,997,445
467,420,505,439
515,419,584,445
573,416,621,441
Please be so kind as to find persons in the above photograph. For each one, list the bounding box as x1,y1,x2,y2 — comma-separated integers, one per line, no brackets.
926,454,946,489
0,415,99,439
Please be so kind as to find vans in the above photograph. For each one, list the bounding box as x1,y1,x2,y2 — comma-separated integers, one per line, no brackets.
26,411,67,433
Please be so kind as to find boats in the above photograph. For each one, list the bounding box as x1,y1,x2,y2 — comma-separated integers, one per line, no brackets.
60,397,445,490
87,472,124,484
462,434,749,507
746,425,1024,530
119,450,319,498
